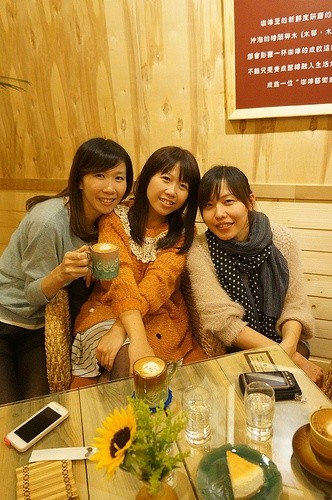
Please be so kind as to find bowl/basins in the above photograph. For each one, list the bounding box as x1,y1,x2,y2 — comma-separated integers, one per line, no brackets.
309,407,332,462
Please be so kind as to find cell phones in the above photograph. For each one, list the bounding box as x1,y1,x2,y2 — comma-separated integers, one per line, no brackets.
6,401,68,453
241,371,295,390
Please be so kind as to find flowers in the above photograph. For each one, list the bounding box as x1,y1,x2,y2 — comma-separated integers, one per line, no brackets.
90,399,190,492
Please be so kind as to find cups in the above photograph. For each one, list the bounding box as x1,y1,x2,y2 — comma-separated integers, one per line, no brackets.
82,242,120,281
133,356,178,408
182,385,212,444
244,381,275,442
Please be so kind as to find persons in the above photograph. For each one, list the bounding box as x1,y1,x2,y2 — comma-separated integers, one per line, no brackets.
70,146,208,389
185,165,324,388
0,138,134,404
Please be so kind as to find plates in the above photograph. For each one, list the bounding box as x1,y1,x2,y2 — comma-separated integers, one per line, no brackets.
292,422,332,482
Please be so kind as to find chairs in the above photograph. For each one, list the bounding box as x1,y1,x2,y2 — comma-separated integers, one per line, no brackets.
45,253,227,395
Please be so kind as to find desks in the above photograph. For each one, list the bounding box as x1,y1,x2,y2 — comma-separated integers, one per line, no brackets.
0,343,332,500
